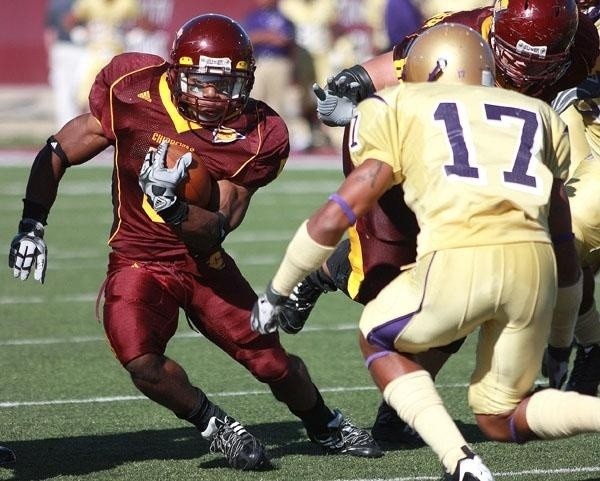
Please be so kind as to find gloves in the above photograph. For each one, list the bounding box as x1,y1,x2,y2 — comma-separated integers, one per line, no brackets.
247,283,284,338
7,216,49,287
309,74,366,129
136,139,194,224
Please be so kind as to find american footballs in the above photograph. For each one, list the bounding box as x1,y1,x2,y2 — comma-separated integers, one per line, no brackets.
165,145,211,209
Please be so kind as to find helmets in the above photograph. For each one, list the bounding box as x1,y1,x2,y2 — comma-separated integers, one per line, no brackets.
165,12,257,128
400,21,498,89
491,1,583,97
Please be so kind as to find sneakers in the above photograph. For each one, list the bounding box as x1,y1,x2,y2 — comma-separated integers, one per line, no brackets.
275,265,338,334
563,342,599,397
198,414,268,471
306,409,386,459
369,414,428,447
